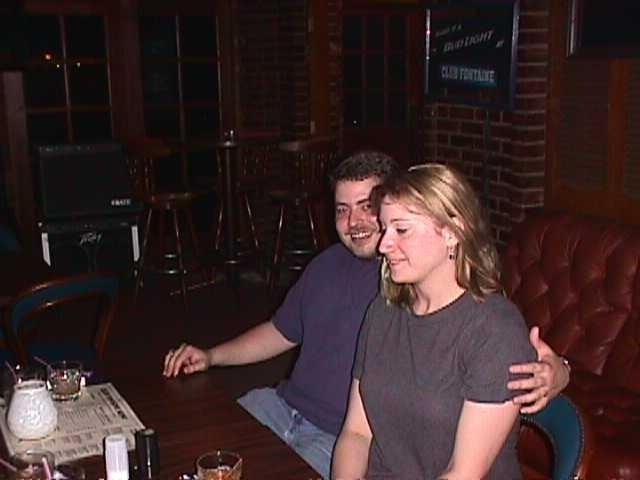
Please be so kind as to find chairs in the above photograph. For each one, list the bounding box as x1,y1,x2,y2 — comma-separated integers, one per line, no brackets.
0,268,121,382
520,392,588,480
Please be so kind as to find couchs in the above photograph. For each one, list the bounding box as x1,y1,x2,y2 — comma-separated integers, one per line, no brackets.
498,210,640,480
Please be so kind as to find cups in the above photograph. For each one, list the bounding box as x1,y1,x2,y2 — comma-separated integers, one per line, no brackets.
195,449,243,480
46,359,82,401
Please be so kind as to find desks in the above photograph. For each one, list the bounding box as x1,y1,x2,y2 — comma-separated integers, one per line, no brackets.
0,371,328,480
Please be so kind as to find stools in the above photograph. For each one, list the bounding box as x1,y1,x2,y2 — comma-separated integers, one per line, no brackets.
130,172,322,313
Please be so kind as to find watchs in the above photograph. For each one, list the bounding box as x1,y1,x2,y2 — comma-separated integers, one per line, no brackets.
560,357,571,375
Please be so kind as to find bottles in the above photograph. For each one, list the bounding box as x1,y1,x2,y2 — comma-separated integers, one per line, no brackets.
103,426,163,480
7,380,57,440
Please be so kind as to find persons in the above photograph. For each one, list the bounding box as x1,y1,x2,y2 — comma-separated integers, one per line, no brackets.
330,164,538,479
163,151,571,479
